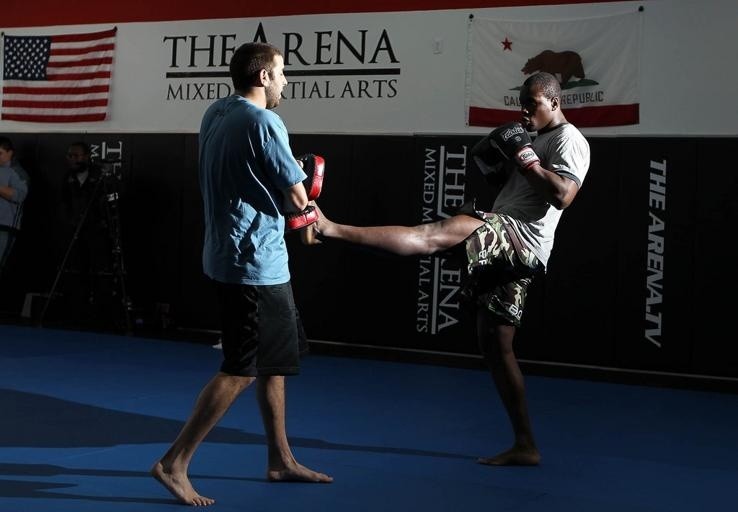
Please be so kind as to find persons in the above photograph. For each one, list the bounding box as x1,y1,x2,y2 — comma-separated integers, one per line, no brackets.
293,72,593,466
59,142,113,320
0,141,30,257
152,42,335,506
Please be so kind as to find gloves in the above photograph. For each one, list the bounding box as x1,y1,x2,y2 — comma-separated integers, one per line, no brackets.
487,121,542,177
468,136,506,178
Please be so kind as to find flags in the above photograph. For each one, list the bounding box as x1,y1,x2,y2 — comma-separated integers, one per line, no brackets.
464,10,644,126
0,25,117,124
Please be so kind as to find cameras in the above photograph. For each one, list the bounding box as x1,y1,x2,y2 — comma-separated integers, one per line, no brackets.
75,159,122,173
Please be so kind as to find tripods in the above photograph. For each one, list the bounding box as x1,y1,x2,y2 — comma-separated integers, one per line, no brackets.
39,176,130,325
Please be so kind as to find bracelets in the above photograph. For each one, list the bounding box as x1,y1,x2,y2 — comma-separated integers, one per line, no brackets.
516,148,540,170
